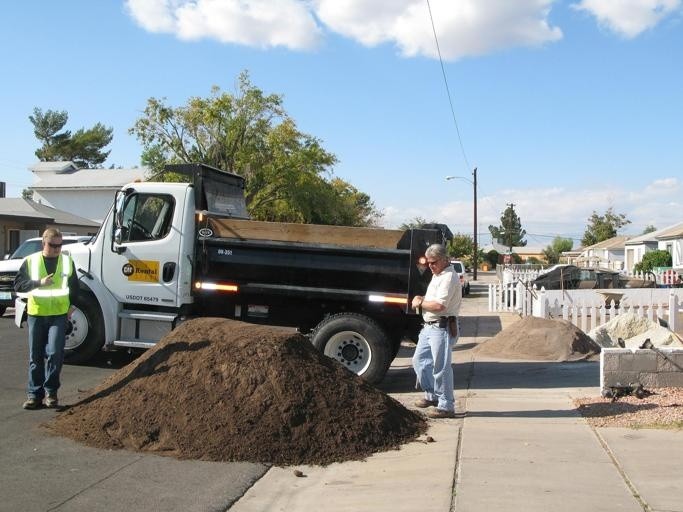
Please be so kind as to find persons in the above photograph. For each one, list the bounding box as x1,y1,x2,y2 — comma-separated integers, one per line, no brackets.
13,228,80,409
411,244,463,418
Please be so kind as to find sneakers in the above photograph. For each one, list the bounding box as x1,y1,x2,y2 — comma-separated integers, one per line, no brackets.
45,396,58,408
22,398,43,410
427,407,455,418
415,398,438,408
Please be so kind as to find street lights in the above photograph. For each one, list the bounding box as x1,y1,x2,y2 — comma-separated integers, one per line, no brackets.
445,166,478,279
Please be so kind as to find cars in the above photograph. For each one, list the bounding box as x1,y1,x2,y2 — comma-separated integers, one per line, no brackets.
449,258,469,295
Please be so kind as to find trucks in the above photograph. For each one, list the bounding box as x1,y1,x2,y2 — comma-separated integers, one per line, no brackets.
12,165,453,382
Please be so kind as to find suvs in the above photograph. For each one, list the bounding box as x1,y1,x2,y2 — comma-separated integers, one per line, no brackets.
0,235,90,316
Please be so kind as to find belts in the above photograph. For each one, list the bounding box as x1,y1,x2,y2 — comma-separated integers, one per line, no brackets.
426,321,438,325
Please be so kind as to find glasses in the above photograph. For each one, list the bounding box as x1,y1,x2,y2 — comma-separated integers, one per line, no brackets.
47,243,62,248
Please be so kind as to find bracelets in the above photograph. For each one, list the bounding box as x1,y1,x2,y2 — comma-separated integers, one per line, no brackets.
419,299,422,307
69,304,76,310
